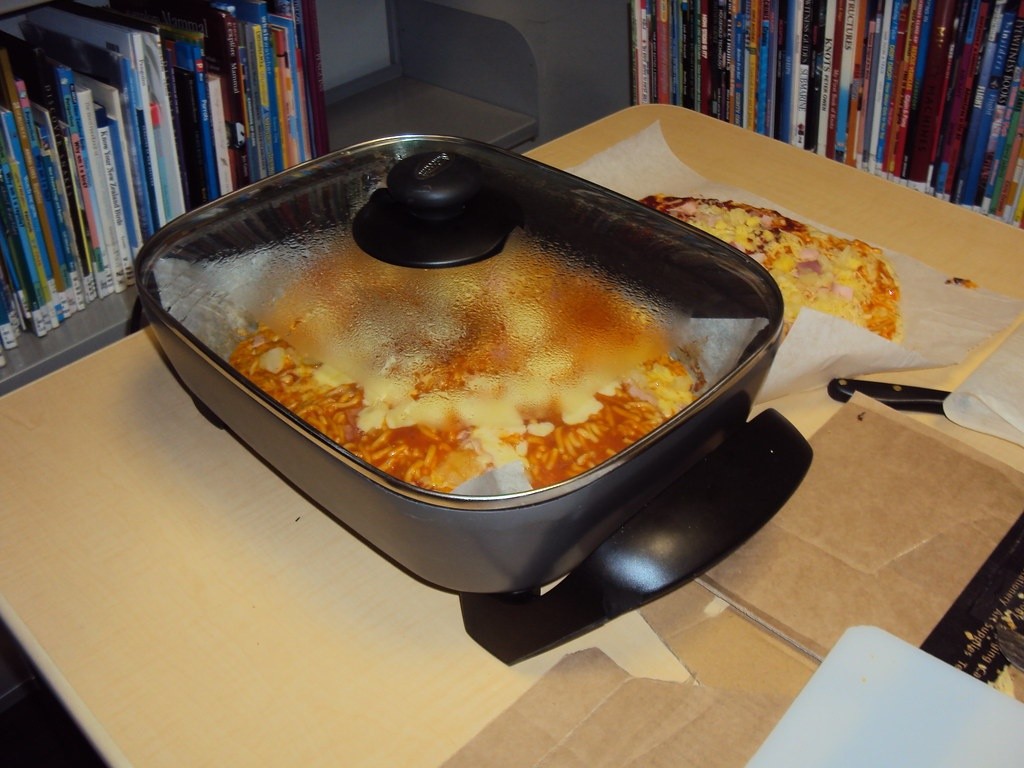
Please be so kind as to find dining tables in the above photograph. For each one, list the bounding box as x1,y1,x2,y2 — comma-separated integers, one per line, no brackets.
0,102,1024,768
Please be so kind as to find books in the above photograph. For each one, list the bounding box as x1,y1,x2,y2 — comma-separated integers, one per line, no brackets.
632,0,1024,230
0,0,330,368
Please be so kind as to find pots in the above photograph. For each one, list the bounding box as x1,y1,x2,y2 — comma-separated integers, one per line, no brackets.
136,133,813,667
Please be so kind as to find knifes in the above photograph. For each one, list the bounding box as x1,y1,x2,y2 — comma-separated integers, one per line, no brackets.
828,378,1024,427
